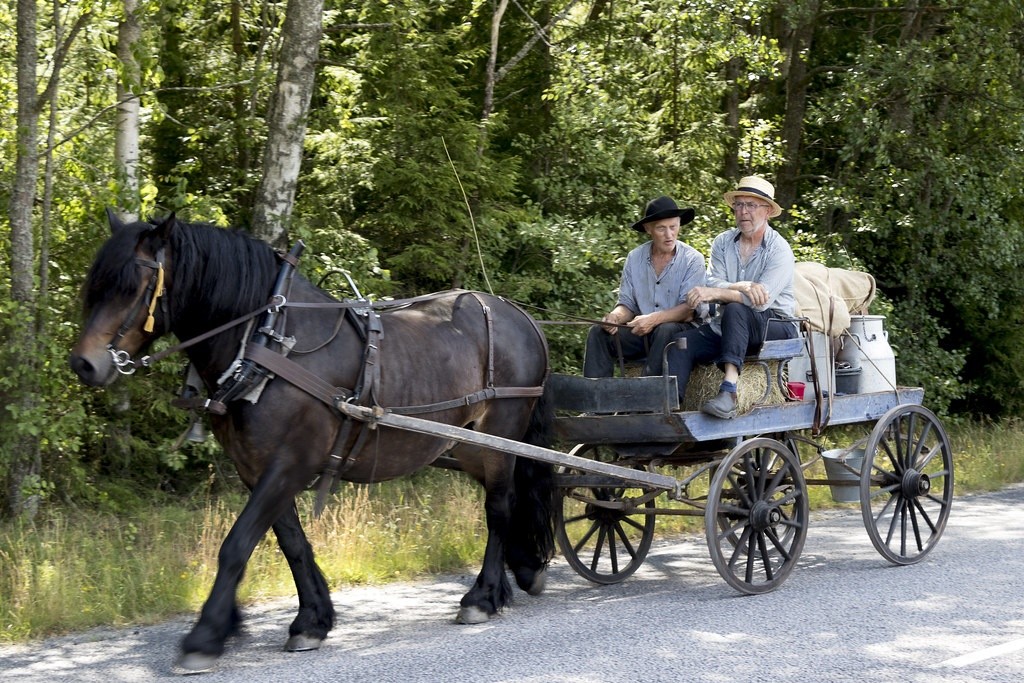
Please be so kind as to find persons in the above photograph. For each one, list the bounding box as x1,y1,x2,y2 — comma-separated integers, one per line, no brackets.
577,197,706,416
666,177,799,421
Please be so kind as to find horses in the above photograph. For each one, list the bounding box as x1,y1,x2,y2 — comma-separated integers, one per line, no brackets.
70,204,559,675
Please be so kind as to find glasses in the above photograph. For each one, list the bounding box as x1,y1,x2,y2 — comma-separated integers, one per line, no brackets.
731,201,770,212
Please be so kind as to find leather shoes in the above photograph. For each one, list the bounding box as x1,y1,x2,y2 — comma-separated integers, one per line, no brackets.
702,390,740,420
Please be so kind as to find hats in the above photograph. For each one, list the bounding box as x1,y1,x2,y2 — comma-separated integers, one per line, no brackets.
631,196,696,232
724,176,782,217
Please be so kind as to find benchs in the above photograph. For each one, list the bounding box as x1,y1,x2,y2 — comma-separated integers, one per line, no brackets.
744,318,809,406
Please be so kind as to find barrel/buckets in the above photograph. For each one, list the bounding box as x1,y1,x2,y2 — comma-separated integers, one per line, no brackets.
837,314,897,394
820,449,865,503
786,382,805,401
835,368,862,394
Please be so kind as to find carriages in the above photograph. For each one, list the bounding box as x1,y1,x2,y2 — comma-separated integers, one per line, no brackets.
65,199,958,678
